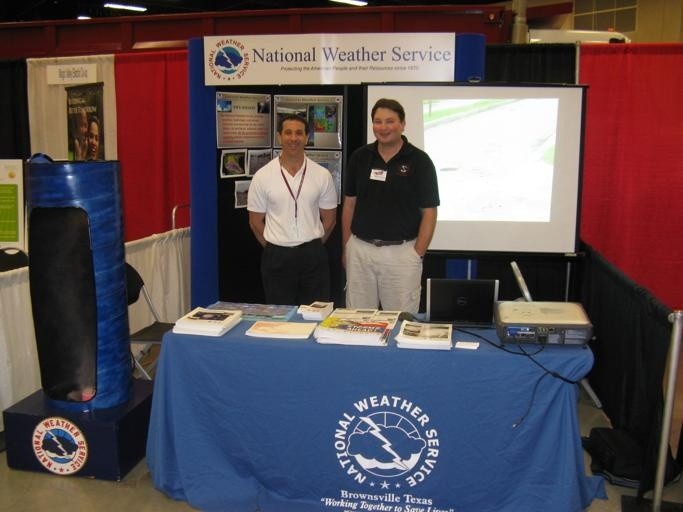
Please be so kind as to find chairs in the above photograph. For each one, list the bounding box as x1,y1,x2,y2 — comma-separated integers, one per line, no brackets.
124,261,174,379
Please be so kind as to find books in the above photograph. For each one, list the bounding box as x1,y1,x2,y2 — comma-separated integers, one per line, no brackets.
173,300,454,350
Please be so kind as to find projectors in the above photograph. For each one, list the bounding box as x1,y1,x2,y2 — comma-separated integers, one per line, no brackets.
494,300,593,346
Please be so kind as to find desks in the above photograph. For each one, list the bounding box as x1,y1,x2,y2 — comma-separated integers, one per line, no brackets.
145,301,605,512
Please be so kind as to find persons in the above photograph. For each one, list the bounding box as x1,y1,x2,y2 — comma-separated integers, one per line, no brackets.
247,114,338,304
72,109,103,161
338,98,439,312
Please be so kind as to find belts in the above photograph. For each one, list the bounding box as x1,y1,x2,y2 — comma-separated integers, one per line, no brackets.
356,235,404,246
